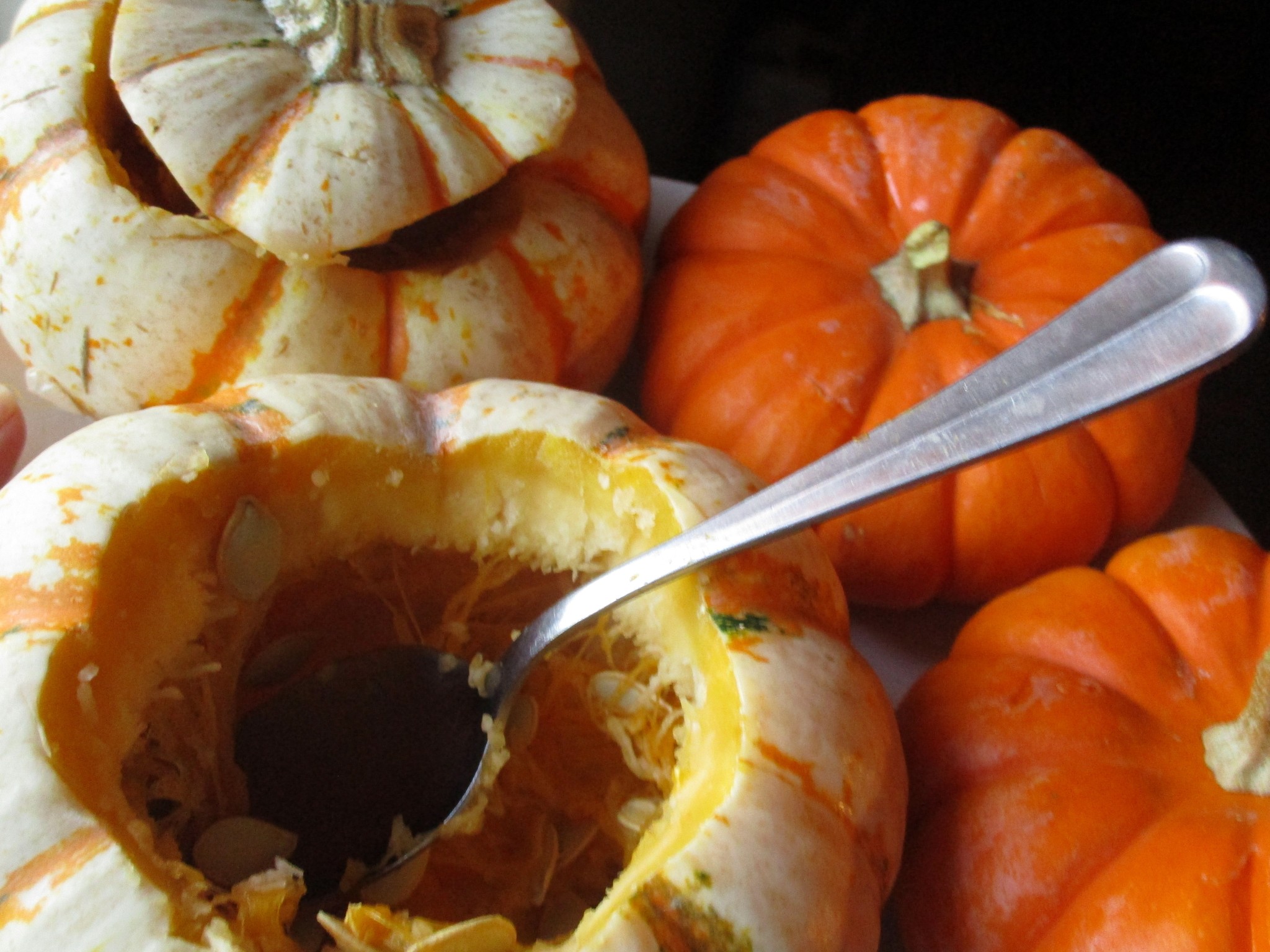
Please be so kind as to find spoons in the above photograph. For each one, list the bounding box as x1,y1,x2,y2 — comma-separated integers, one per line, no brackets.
234,238,1269,903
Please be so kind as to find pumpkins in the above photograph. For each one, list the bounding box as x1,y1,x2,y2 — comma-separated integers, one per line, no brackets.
0,0,903,952
878,518,1270,950
642,92,1204,616
